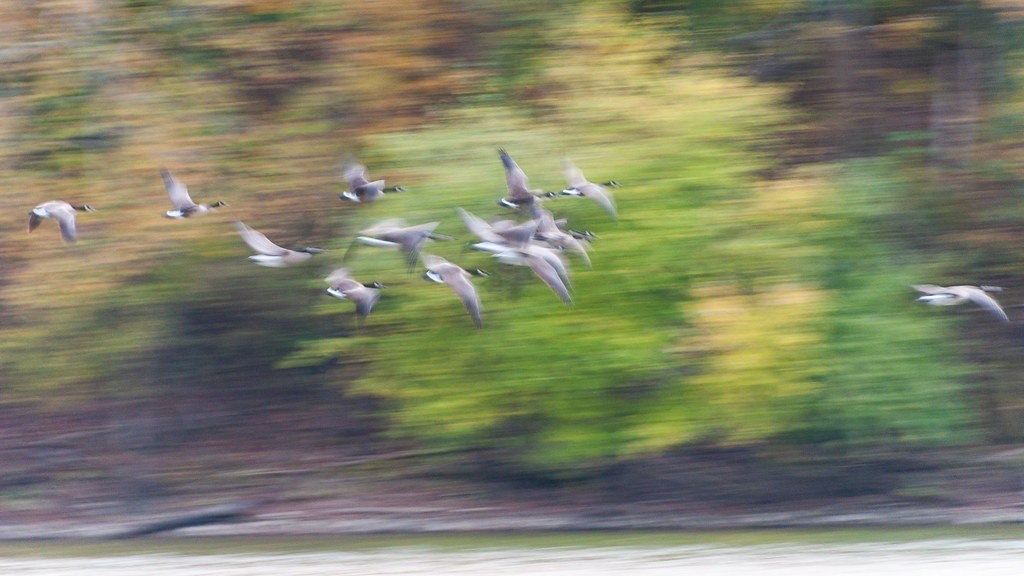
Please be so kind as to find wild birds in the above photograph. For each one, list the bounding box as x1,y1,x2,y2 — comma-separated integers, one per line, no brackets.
25,142,624,331
908,277,1010,327
